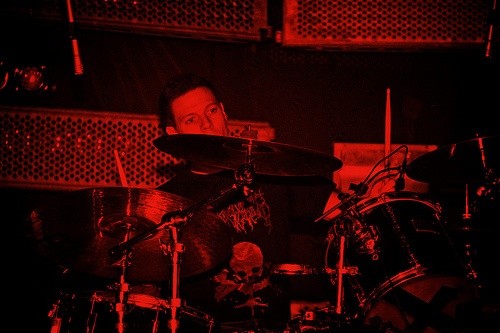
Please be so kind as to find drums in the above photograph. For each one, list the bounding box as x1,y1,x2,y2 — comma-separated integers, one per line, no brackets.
323,191,477,333
47,289,215,333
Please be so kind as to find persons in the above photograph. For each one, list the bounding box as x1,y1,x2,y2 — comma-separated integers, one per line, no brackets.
128,72,273,333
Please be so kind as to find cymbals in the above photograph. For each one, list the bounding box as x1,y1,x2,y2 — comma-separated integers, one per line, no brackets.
152,133,344,177
37,186,230,282
406,135,500,187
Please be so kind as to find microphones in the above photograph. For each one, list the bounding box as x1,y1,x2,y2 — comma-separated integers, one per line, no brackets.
395,157,407,190
0,61,43,92
65,0,84,76
484,0,500,59
